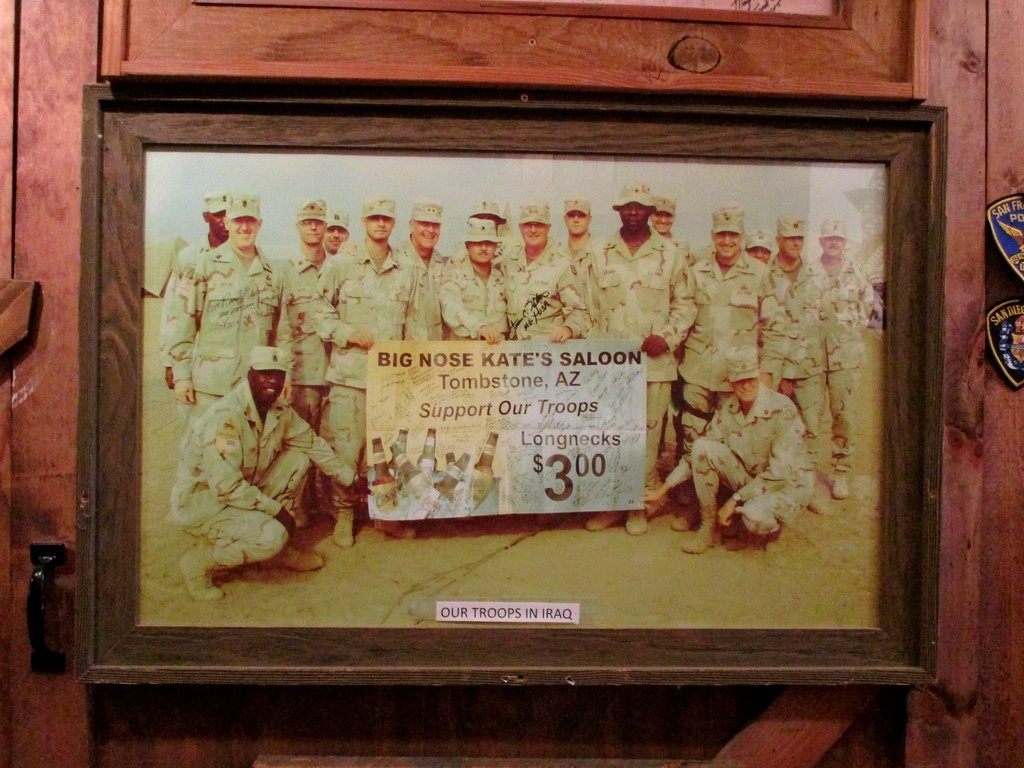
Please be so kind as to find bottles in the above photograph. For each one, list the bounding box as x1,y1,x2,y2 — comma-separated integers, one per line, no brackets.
372,438,398,513
444,452,467,505
417,428,437,480
390,444,431,498
407,453,471,520
450,431,498,518
387,427,408,486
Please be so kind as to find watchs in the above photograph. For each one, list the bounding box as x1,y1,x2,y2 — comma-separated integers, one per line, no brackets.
733,492,741,504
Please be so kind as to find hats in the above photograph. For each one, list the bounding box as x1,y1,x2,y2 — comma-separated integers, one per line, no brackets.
613,180,656,215
564,199,591,215
411,200,442,223
203,192,231,213
326,211,350,236
226,193,260,221
362,197,395,219
250,346,287,372
464,217,502,243
820,218,847,240
778,216,807,236
470,200,506,224
711,208,744,234
745,230,772,252
519,202,551,226
652,195,675,216
297,200,326,222
724,345,758,382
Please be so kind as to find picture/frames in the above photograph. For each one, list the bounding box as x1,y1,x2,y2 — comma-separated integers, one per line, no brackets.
72,82,948,689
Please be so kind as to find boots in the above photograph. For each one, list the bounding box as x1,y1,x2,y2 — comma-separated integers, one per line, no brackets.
179,490,416,600
584,465,849,553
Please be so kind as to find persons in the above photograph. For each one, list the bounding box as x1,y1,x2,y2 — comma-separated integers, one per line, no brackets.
641,350,815,554
158,180,874,549
171,346,375,600
